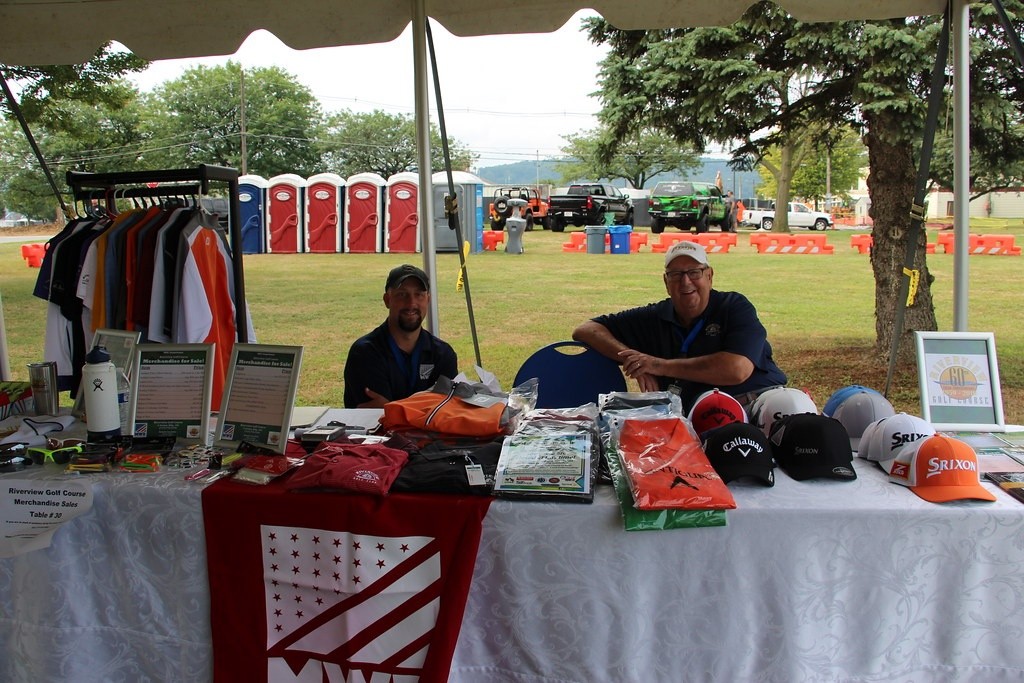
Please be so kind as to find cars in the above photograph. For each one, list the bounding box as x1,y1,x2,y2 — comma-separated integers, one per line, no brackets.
0,212,23,227
16,214,48,226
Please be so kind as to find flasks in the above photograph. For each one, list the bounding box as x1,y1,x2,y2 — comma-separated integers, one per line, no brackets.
27,361,59,417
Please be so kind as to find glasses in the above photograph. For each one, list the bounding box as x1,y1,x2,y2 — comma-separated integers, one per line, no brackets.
665,266,709,282
0,437,88,473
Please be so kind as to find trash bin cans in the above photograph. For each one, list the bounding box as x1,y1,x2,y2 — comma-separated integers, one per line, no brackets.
584,225,608,254
608,225,632,254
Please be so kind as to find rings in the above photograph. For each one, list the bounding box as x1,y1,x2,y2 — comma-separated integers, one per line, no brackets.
636,361,639,366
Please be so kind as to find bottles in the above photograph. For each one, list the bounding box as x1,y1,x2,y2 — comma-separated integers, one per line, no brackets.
116,368,130,436
81,346,120,443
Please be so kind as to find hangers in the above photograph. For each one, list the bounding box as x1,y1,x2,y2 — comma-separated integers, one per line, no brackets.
45,182,212,253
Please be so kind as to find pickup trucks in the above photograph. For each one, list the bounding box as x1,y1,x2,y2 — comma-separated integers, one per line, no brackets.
743,201,833,231
548,182,635,233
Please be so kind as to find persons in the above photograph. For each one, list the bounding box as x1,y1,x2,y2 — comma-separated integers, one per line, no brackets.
572,240,787,423
725,190,738,233
343,264,458,410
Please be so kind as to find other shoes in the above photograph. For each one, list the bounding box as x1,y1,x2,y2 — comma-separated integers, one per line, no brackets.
732,231,737,233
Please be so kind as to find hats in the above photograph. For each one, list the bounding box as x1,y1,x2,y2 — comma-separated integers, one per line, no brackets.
687,385,997,502
385,264,430,293
664,241,710,272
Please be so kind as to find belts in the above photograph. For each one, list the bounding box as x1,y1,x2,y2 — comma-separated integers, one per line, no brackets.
734,387,784,405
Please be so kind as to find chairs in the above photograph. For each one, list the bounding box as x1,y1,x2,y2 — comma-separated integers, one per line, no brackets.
595,188,600,195
509,341,628,412
519,194,527,200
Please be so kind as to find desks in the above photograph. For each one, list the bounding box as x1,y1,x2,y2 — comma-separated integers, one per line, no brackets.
0,406,1023,683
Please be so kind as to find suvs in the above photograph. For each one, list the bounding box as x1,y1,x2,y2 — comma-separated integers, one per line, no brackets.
488,183,553,232
649,181,733,234
